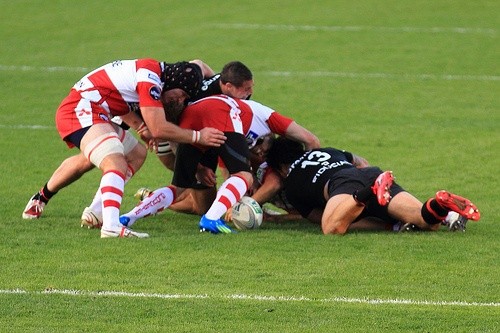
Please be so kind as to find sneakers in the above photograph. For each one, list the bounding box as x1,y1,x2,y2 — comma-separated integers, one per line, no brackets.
135,188,151,202
444,212,467,231
398,223,415,233
101,225,148,239
435,190,481,221
371,171,395,205
118,216,131,228
199,215,233,235
23,194,45,219
81,207,103,229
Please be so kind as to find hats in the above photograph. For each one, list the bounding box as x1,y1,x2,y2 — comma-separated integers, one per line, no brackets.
162,61,203,98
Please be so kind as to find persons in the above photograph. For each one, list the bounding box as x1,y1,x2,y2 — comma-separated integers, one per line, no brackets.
22,60,480,235
55,58,227,238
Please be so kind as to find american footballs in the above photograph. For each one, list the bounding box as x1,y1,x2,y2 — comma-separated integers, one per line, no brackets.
231,195,264,232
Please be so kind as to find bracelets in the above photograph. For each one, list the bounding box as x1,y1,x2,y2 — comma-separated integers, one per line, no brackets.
192,130,200,142
136,121,147,134
